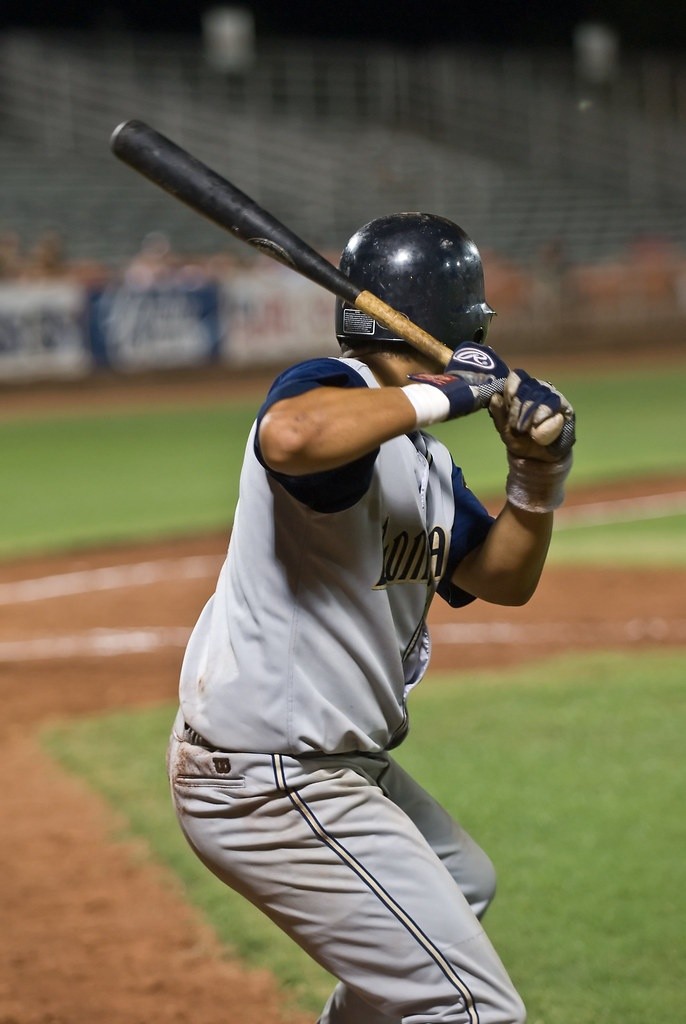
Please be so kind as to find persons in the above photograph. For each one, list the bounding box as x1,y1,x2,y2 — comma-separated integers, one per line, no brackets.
170,212,574,1024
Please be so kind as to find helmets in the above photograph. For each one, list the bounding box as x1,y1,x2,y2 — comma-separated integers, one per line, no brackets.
335,212,497,350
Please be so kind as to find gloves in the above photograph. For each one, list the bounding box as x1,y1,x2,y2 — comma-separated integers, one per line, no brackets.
406,340,511,423
489,368,576,465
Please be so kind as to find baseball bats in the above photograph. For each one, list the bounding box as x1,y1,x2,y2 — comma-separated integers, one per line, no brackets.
108,116,455,369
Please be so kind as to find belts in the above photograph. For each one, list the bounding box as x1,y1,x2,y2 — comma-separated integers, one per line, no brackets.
183,722,205,748
